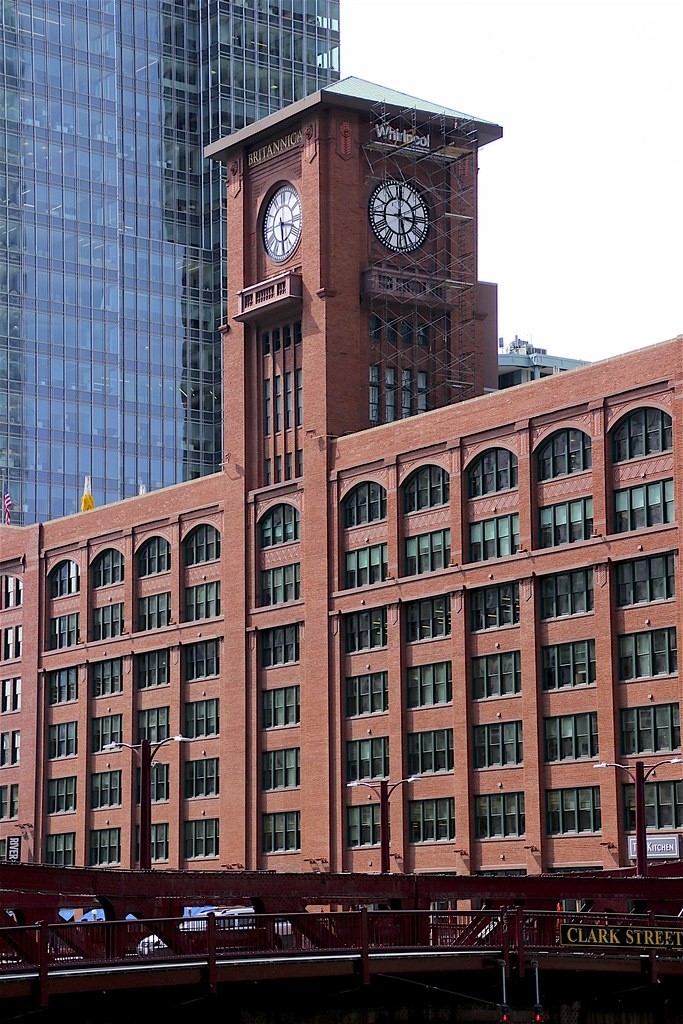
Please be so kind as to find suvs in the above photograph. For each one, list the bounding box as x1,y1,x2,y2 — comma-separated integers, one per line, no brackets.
137,906,294,958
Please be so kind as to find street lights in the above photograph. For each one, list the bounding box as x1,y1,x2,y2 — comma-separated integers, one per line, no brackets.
100,736,194,868
592,759,683,878
345,778,424,874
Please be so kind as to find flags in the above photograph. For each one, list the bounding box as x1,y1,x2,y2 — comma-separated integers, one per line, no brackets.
4,482,12,525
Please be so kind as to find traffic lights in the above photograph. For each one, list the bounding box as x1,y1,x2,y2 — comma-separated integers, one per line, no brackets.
534,1004,544,1024
500,1005,511,1024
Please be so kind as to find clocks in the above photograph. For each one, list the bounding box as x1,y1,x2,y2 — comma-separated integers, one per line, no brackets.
369,180,430,252
262,186,302,262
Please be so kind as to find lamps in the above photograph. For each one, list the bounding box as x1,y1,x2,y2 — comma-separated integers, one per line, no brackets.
221,863,244,869
448,563,458,567
591,533,602,538
454,849,468,855
389,852,403,860
385,577,395,580
303,858,328,864
600,841,617,849
516,549,528,552
523,845,539,852
